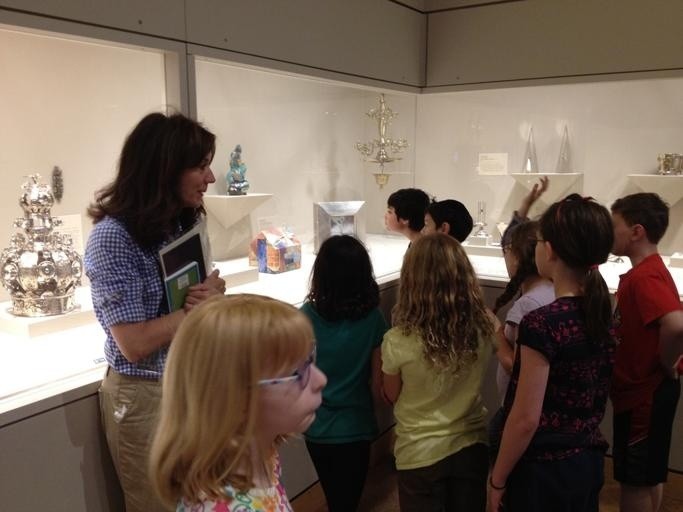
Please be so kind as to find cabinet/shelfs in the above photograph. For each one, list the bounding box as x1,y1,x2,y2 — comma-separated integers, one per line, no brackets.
0,392,124,510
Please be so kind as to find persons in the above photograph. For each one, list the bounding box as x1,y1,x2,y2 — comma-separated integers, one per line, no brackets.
82,113,227,510
380,233,495,511
296,234,391,511
480,172,556,407
611,192,683,511
147,293,328,510
420,199,473,244
383,188,431,248
486,187,610,511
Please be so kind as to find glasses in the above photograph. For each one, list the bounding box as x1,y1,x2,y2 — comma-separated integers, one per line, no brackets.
502,247,517,254
244,346,317,389
530,236,550,247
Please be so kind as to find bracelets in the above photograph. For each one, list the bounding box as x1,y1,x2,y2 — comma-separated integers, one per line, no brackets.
488,478,508,495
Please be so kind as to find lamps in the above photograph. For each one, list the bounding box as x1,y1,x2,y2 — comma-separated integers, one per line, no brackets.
356,92,409,191
